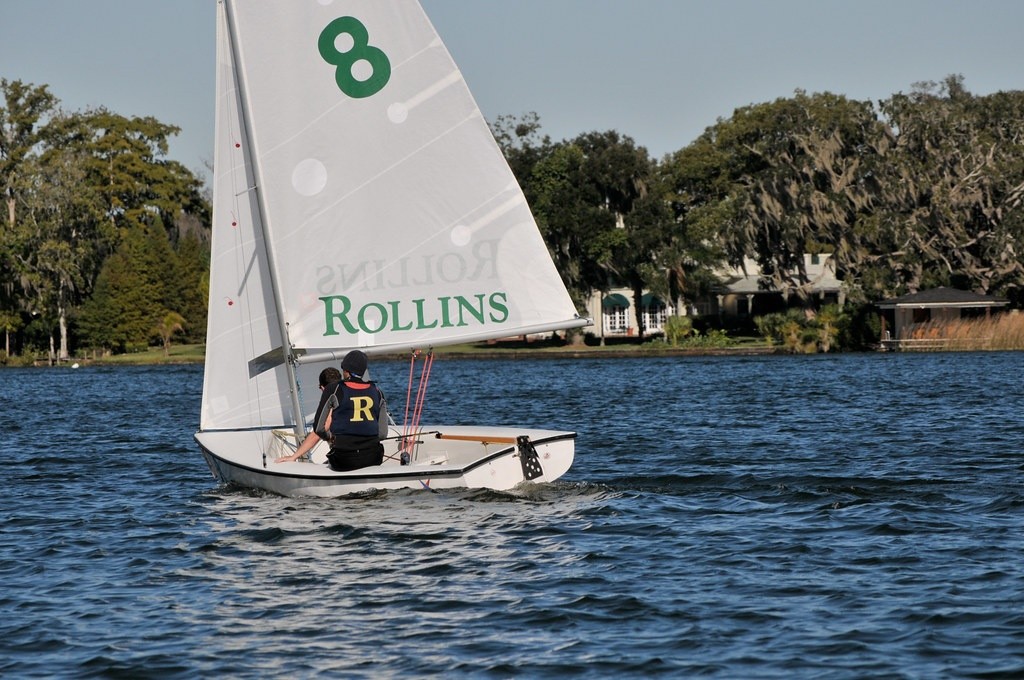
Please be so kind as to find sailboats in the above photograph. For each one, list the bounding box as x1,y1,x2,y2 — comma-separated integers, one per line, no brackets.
197,0,594,509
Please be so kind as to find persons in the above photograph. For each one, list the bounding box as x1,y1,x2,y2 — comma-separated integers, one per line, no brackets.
313,350,389,473
273,367,342,464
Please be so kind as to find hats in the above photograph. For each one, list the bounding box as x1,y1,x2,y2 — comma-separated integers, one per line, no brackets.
341,350,369,377
